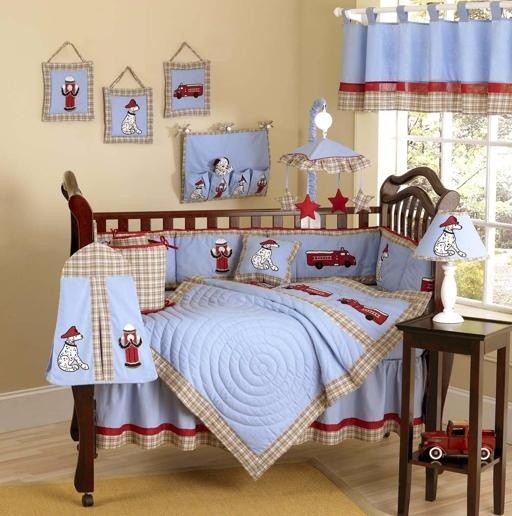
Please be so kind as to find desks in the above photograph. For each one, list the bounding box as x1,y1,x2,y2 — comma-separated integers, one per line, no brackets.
395,312,512,516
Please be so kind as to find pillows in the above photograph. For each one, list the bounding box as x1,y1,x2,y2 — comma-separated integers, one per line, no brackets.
233,233,303,286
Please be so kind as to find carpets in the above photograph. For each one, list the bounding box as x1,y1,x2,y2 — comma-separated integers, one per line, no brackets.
0,456,396,516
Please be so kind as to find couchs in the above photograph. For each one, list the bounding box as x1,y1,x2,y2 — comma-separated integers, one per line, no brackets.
61,166,462,506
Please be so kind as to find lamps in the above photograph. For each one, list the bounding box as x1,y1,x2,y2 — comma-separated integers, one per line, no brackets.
276,96,372,202
412,210,488,323
